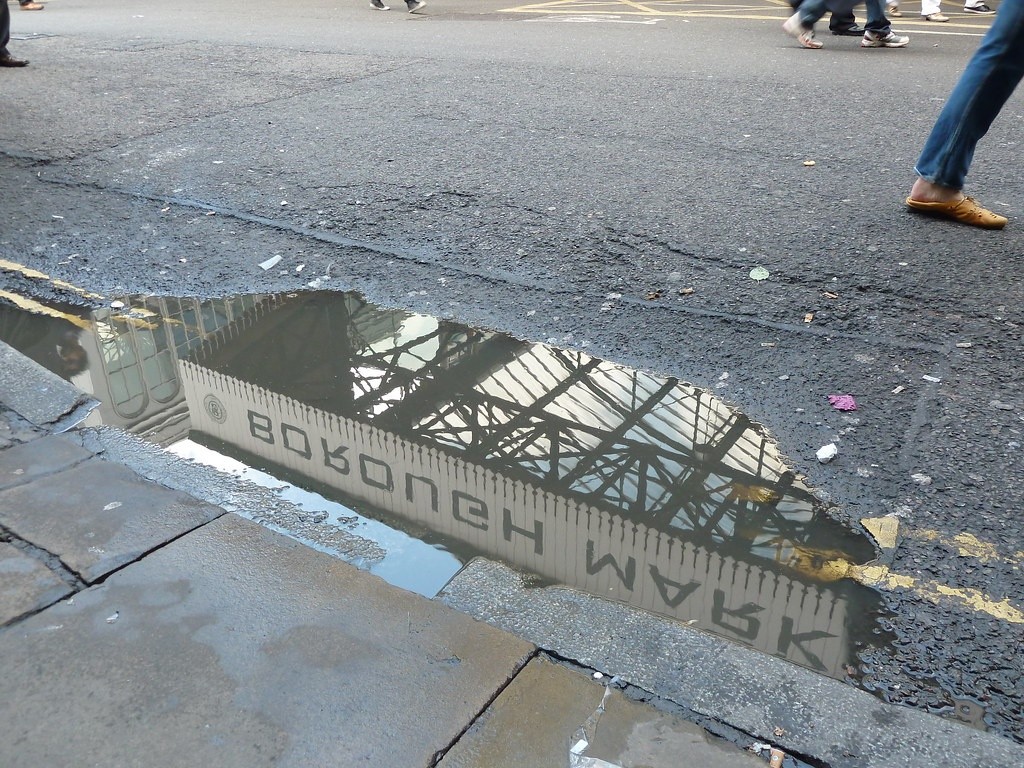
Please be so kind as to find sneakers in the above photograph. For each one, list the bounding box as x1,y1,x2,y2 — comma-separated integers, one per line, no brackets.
964,4,996,14
860,29,910,47
782,11,823,49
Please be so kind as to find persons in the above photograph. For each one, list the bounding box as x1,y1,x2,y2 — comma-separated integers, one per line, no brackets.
0,0,44,68
369,0,427,13
783,0,996,49
905,0,1024,229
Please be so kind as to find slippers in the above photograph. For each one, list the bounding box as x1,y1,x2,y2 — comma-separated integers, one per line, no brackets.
905,192,1008,229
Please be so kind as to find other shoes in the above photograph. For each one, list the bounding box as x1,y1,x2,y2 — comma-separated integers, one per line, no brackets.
369,2,390,11
19,3,44,10
926,12,949,22
885,3,902,17
831,25,866,35
0,54,30,67
406,0,426,12
